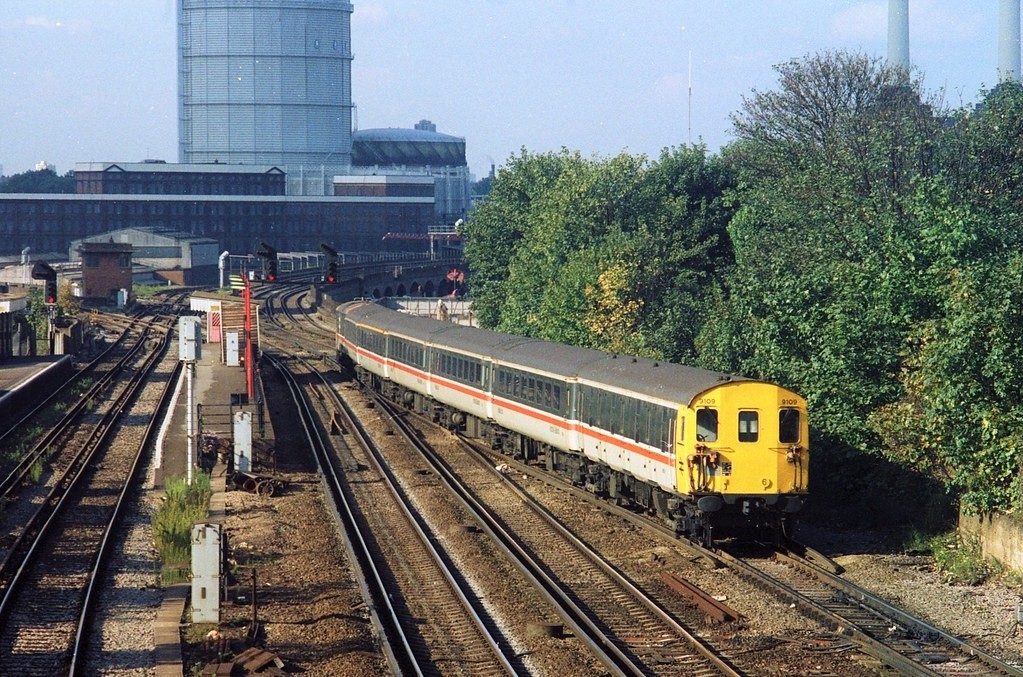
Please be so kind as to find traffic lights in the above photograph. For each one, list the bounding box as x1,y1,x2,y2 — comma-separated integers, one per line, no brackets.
268,259,276,282
328,262,338,282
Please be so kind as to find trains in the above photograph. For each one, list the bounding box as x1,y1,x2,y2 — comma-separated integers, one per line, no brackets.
337,300,808,553
